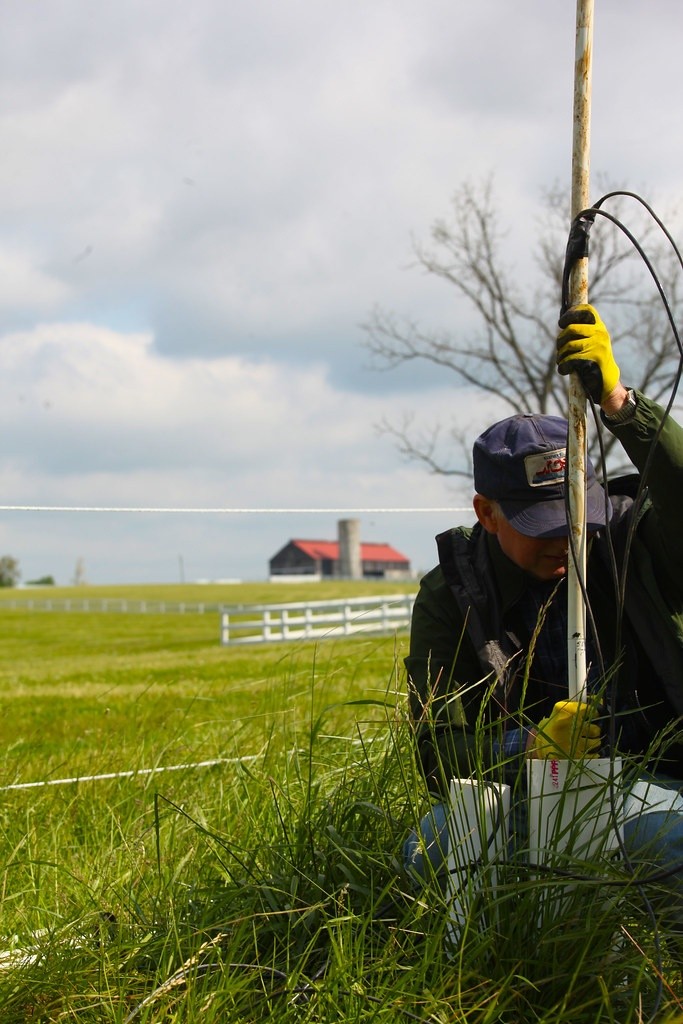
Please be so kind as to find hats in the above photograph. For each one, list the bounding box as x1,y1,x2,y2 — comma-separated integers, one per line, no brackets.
473,414,614,537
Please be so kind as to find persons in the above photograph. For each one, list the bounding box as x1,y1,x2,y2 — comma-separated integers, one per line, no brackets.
402,303,683,898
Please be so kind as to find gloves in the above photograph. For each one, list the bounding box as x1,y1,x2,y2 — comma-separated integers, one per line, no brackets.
535,701,602,759
552,303,621,403
